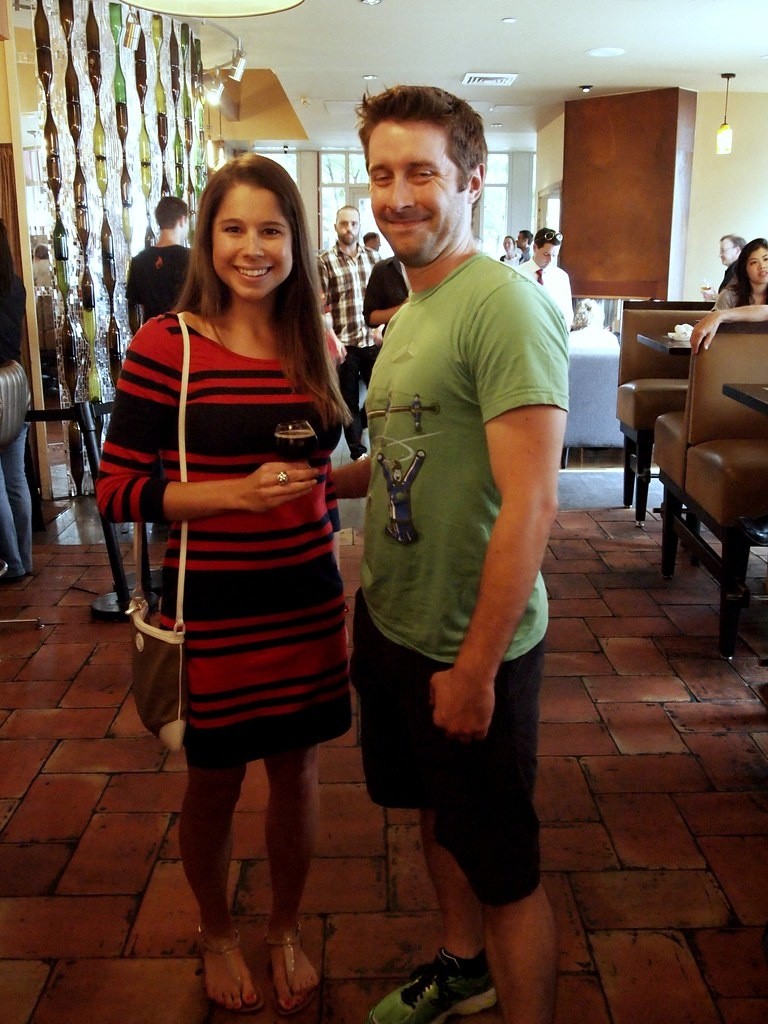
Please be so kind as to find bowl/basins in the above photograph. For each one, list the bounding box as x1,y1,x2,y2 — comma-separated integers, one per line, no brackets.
669,333,690,346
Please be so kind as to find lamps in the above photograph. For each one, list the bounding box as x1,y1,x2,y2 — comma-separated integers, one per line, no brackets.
198,18,248,106
716,73,736,155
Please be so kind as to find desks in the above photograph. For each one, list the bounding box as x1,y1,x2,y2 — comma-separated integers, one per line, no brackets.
636,330,691,356
721,383,768,415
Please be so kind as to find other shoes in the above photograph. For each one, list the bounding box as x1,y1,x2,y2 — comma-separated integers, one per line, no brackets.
0,559,8,576
0,574,25,584
745,528,768,545
356,453,368,462
361,427,370,448
25,571,32,576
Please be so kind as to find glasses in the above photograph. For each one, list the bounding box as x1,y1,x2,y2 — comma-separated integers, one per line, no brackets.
534,231,564,243
719,244,738,256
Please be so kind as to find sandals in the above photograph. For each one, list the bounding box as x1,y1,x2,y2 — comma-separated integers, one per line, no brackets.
197,924,263,1014
264,917,318,1014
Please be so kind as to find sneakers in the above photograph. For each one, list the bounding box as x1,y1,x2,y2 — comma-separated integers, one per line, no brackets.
366,950,496,1024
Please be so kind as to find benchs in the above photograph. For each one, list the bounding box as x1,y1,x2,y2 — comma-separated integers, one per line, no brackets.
615,301,768,657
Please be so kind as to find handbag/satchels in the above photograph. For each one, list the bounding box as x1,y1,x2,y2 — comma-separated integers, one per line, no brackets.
126,597,189,754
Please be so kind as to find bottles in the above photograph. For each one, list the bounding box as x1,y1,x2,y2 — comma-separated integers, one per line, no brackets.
33,0,205,501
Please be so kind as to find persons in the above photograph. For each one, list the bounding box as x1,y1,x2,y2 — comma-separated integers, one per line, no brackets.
327,83,569,1024
126,194,201,337
317,203,414,461
90,152,350,1018
499,226,620,356
0,216,35,581
689,235,768,353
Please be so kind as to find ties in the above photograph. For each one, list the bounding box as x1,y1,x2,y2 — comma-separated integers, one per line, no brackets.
536,268,544,286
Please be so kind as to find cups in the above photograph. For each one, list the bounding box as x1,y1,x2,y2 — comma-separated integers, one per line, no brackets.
700,278,711,293
273,421,320,462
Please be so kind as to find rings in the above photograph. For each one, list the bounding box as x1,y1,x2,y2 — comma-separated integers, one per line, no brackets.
278,471,289,485
